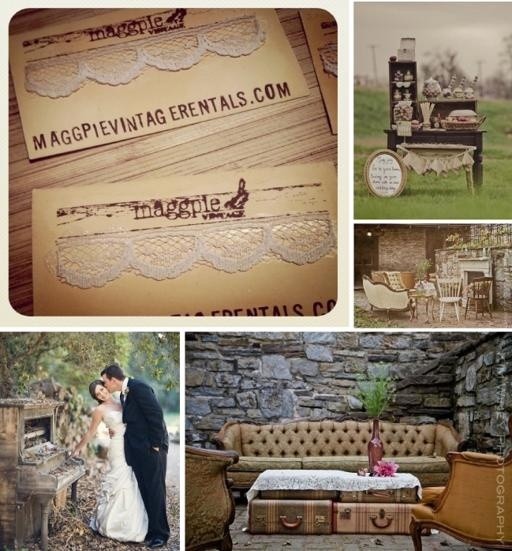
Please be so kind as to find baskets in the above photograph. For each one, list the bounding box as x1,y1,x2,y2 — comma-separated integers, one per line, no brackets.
441,112,487,131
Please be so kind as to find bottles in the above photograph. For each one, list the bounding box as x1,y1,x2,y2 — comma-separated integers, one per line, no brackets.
368,418,383,474
422,77,441,99
394,101,412,125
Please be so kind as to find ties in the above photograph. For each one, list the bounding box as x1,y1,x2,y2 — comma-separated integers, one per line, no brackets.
120,392,124,405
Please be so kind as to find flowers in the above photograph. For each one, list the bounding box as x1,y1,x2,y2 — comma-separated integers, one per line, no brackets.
371,459,400,479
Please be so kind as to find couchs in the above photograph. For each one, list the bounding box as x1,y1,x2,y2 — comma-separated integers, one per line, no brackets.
209,414,463,506
361,269,415,323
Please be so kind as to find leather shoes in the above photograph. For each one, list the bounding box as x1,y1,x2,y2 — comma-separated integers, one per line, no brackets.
148,538,167,549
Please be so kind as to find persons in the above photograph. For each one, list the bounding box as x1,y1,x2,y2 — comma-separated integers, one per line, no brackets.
70,365,171,549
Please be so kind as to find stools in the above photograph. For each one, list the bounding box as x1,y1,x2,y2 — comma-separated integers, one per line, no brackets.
395,141,477,197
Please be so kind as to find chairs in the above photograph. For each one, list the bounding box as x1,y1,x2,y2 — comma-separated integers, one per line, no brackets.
464,276,494,321
185,444,239,551
381,270,417,318
434,273,464,323
409,413,512,551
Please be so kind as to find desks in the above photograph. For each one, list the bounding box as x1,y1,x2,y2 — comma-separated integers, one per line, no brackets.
382,128,489,187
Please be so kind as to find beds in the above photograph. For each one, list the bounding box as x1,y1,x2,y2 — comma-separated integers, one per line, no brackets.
407,288,437,324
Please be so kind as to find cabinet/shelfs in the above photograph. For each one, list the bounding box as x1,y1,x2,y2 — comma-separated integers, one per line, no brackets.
0,396,88,551
387,60,418,129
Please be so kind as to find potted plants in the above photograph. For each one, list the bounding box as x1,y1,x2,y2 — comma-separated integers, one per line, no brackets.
344,355,401,476
414,258,434,291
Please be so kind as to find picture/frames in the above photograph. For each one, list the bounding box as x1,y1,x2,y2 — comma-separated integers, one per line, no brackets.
363,149,408,199
